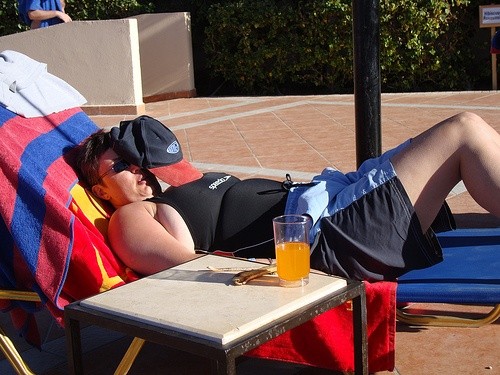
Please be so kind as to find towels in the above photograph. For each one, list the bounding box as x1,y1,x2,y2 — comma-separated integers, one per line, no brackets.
66,181,399,373
0,49,88,118
0,102,105,352
18,0,64,28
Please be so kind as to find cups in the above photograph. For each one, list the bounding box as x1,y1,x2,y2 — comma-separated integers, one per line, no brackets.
273,215,311,287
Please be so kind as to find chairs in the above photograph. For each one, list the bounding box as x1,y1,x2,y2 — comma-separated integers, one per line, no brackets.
0,49,500,375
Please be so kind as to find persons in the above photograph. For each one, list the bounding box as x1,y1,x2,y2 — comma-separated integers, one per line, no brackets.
74,111,500,282
18,0,72,29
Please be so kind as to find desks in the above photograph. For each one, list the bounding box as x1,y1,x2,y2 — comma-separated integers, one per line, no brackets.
64,251,370,375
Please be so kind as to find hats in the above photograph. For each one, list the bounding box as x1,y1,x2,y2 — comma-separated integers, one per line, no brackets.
111,115,204,186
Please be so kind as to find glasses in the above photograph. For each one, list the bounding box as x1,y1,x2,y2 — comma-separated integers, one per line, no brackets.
95,160,137,184
